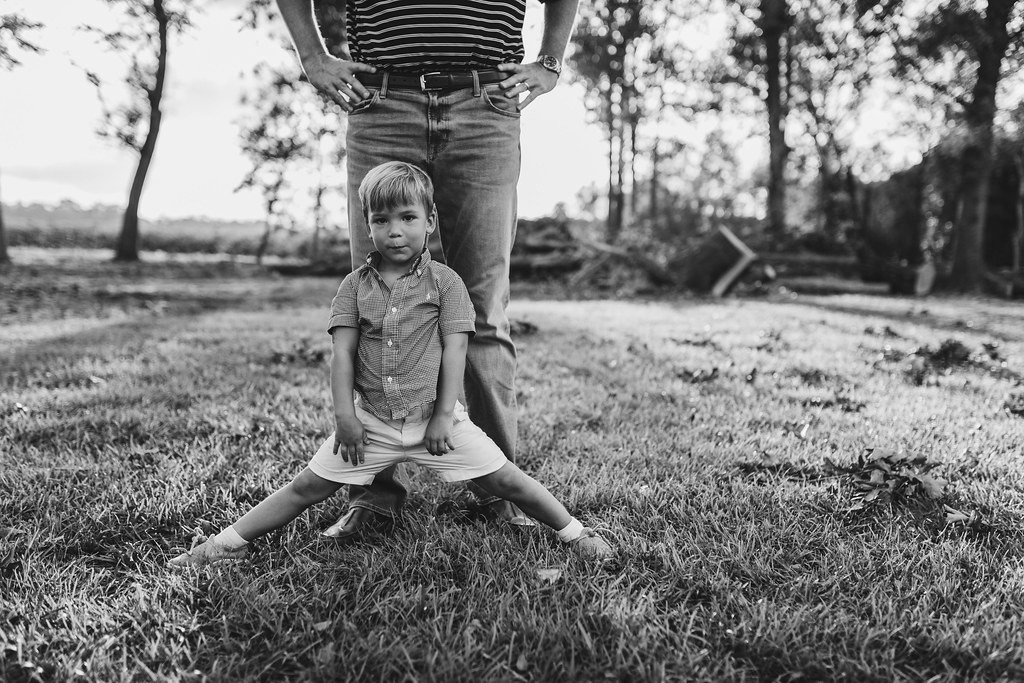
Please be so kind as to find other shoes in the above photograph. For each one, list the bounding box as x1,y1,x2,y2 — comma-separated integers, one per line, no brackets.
168,534,248,570
570,528,612,559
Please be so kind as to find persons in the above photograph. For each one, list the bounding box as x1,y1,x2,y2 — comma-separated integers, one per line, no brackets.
272,0,579,545
165,160,618,576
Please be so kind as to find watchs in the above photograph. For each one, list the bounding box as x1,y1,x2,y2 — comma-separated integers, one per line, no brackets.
536,54,563,78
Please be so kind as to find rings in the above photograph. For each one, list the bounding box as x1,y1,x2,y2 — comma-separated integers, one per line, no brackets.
523,81,532,90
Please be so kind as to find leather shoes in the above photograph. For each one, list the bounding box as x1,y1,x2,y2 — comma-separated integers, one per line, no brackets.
483,498,535,533
321,506,396,547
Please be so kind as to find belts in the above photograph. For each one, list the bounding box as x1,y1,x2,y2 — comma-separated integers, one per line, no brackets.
355,71,516,91
